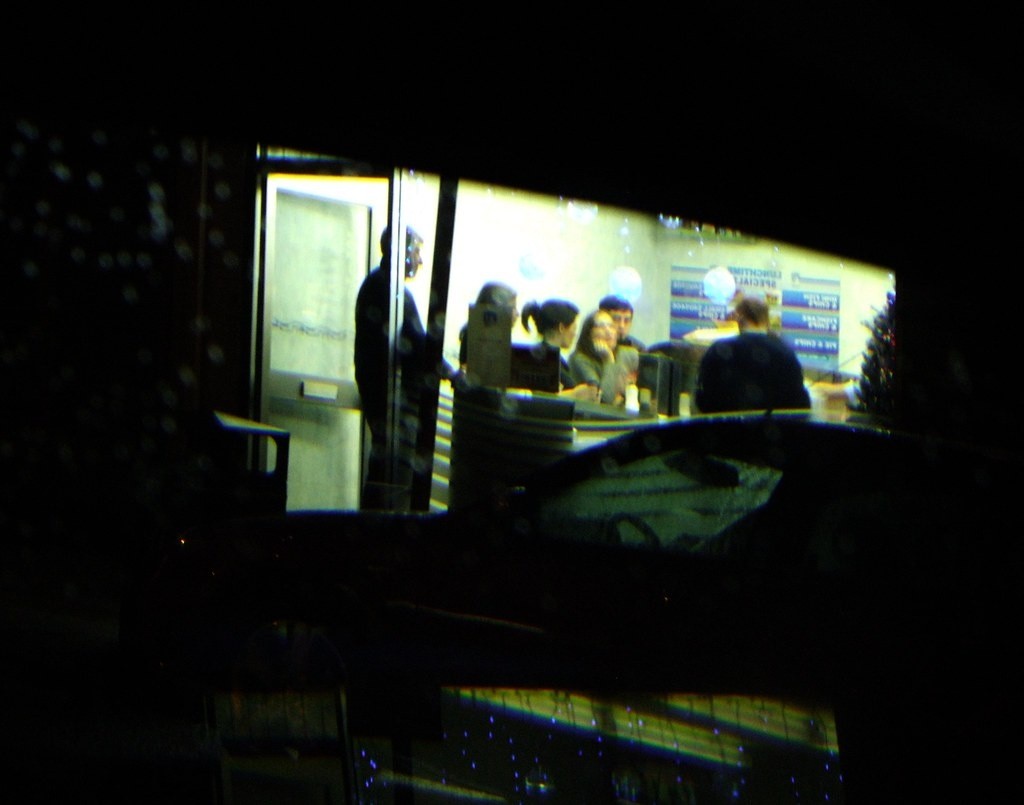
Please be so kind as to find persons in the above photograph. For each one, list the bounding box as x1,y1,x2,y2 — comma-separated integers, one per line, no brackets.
459,281,518,373
696,297,811,414
522,299,603,405
354,222,455,512
598,295,648,386
566,310,635,406
